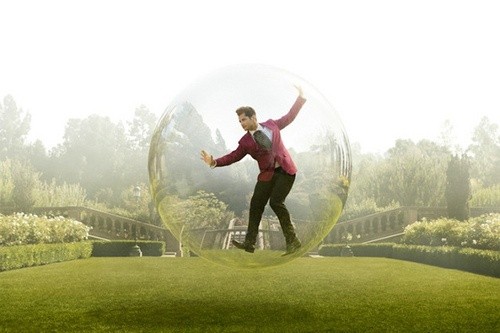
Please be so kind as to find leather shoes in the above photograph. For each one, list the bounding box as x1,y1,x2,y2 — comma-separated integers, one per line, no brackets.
232,239,256,253
281,239,302,257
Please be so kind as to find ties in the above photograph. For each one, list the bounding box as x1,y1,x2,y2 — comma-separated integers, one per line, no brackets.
253,130,272,148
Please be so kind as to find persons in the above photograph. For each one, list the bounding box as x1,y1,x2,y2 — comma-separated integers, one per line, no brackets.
199,83,306,256
